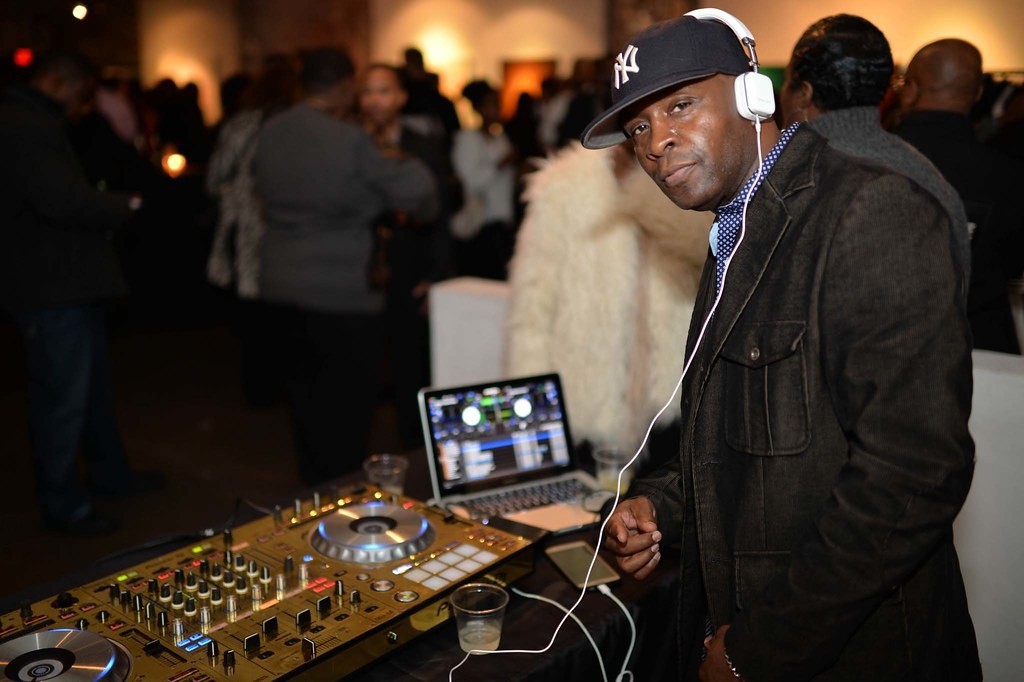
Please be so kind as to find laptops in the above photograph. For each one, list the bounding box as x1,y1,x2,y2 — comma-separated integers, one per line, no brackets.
417,372,619,535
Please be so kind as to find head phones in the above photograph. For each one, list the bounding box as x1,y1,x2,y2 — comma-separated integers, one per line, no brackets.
679,8,778,125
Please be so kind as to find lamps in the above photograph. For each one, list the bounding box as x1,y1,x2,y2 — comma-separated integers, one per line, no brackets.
12,47,36,68
72,3,87,20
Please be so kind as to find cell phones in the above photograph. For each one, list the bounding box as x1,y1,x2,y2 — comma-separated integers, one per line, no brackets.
547,539,617,594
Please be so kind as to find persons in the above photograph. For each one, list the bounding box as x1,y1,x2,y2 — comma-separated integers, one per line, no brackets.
11,14,1023,682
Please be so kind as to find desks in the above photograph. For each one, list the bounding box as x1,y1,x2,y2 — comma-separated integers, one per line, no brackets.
0,446,681,682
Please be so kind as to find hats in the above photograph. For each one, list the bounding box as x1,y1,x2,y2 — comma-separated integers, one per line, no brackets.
581,15,754,150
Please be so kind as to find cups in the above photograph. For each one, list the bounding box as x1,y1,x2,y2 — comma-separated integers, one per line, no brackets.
592,445,639,499
363,455,409,496
450,582,508,655
